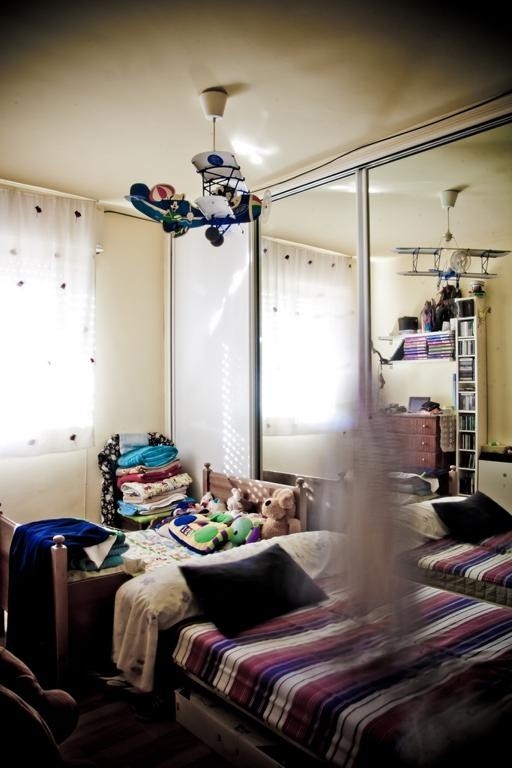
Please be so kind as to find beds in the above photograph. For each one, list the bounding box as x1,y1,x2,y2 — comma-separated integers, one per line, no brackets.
0,459,311,688
109,528,512,768
392,510,512,607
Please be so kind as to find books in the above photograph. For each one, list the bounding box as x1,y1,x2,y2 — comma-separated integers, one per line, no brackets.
402,302,475,495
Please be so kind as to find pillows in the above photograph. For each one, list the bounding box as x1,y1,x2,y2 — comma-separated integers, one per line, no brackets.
176,541,332,638
431,489,512,546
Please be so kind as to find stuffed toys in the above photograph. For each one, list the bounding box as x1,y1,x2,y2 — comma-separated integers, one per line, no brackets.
159,485,302,553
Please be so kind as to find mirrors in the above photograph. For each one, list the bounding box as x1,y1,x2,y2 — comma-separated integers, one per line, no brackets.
259,164,366,534
358,109,511,608
168,217,261,480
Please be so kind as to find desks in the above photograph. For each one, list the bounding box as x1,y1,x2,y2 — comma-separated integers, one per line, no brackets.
384,412,455,476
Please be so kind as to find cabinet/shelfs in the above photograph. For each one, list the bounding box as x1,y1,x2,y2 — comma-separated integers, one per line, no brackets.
452,295,486,497
